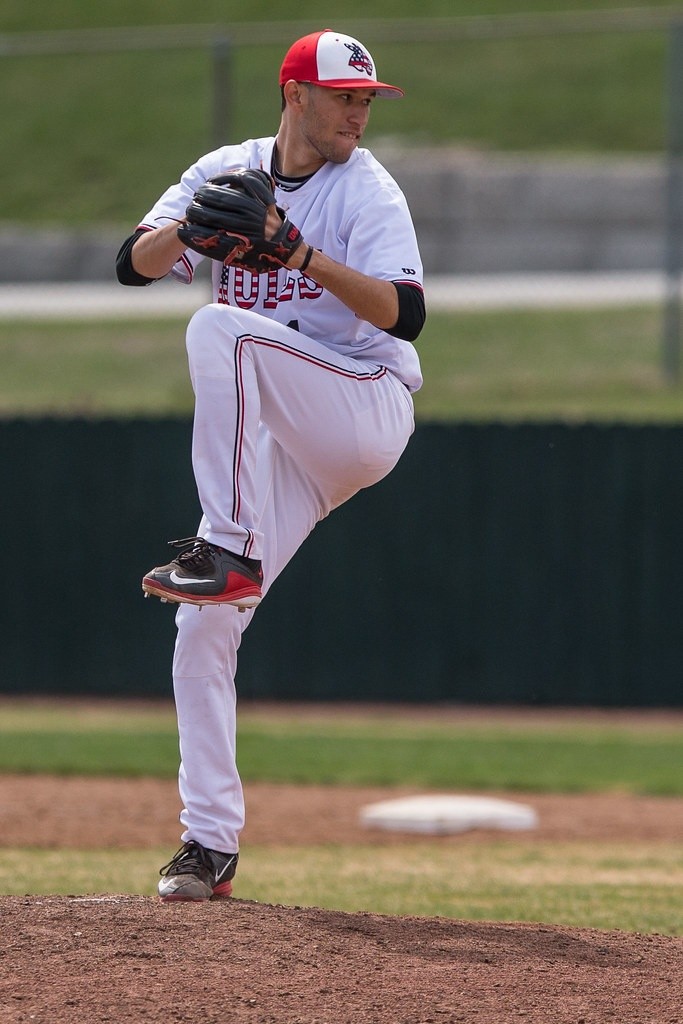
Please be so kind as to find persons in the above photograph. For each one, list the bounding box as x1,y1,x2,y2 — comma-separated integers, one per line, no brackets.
116,28,426,901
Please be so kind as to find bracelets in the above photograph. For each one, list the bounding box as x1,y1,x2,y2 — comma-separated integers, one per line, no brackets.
299,244,314,272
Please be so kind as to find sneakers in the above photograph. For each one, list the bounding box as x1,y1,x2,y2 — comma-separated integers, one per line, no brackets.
157,839,239,902
141,536,264,613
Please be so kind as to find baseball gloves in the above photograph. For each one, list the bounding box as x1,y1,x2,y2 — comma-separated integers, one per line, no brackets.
180,167,305,276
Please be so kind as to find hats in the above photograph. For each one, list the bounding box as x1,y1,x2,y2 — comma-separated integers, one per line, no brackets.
280,29,404,98
